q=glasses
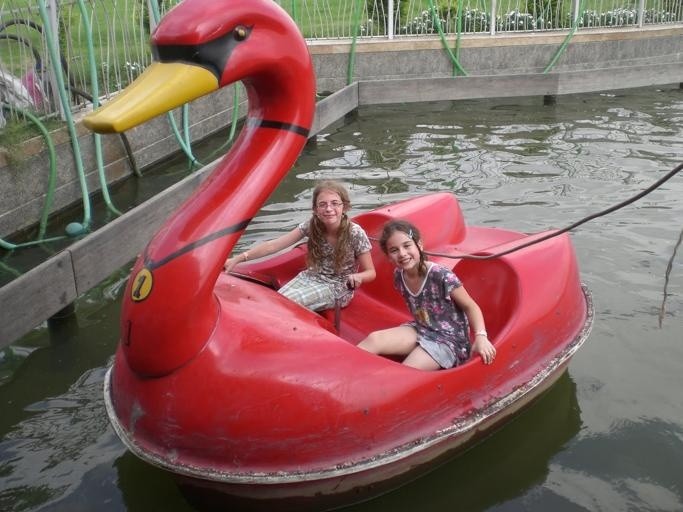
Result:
[315,202,343,209]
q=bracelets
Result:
[474,330,488,336]
[241,251,248,262]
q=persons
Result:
[223,178,378,312]
[356,220,497,368]
